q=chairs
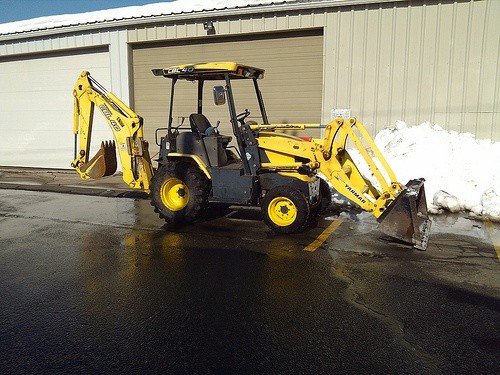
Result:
[189,114,233,147]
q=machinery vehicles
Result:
[69,58,433,253]
